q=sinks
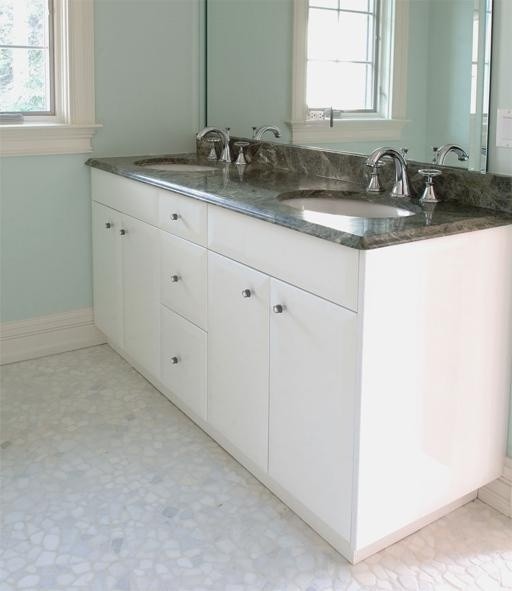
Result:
[276,189,423,218]
[133,156,226,172]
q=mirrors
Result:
[204,0,494,177]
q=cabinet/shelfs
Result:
[90,166,511,565]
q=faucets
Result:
[252,124,281,140]
[195,127,232,162]
[432,144,470,165]
[363,145,413,196]
